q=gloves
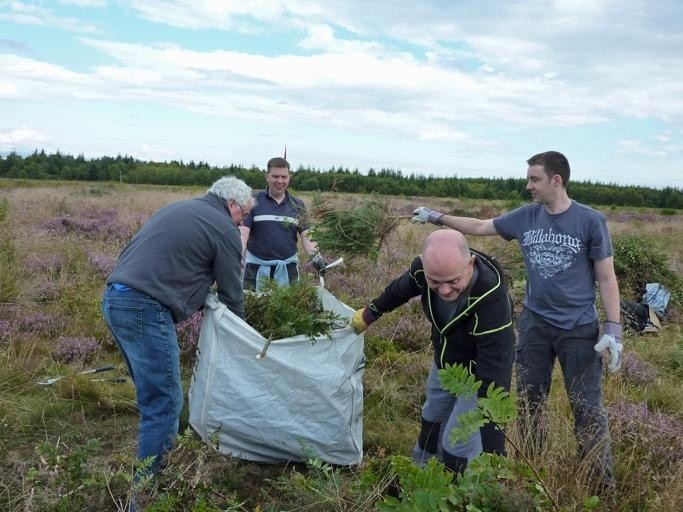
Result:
[593,321,623,374]
[411,207,443,226]
[309,251,330,277]
[351,305,379,335]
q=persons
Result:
[348,227,518,473]
[226,157,324,300]
[98,172,259,491]
[409,150,626,501]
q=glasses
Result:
[233,198,248,220]
